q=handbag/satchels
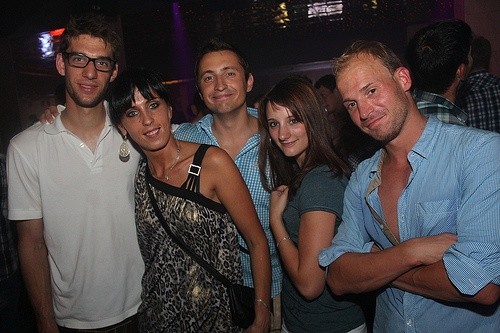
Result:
[227,283,258,328]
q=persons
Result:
[405,18,500,134]
[6,15,180,333]
[189,91,209,124]
[319,40,500,333]
[0,153,40,331]
[252,96,269,108]
[315,75,369,164]
[260,76,368,333]
[39,41,285,333]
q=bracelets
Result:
[276,235,293,247]
[256,296,270,312]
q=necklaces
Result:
[147,139,180,182]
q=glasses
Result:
[61,50,117,72]
